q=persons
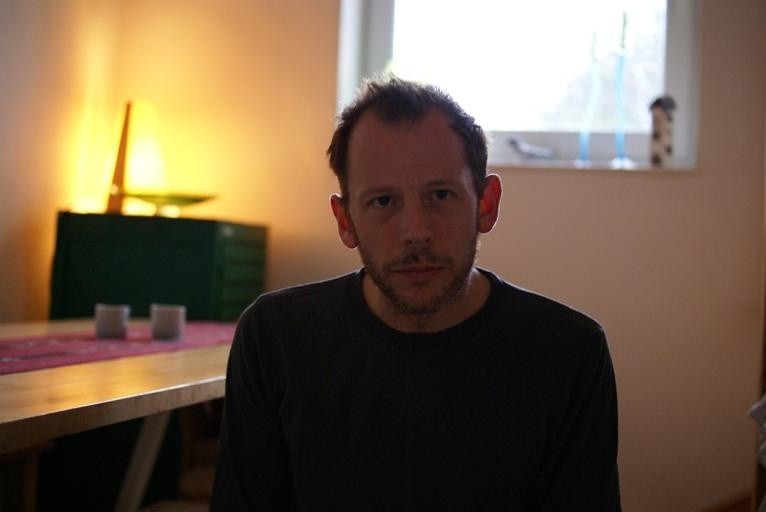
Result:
[209,77,620,512]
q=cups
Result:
[93,304,129,337]
[151,302,186,339]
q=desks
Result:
[1,314,235,512]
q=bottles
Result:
[650,96,676,164]
